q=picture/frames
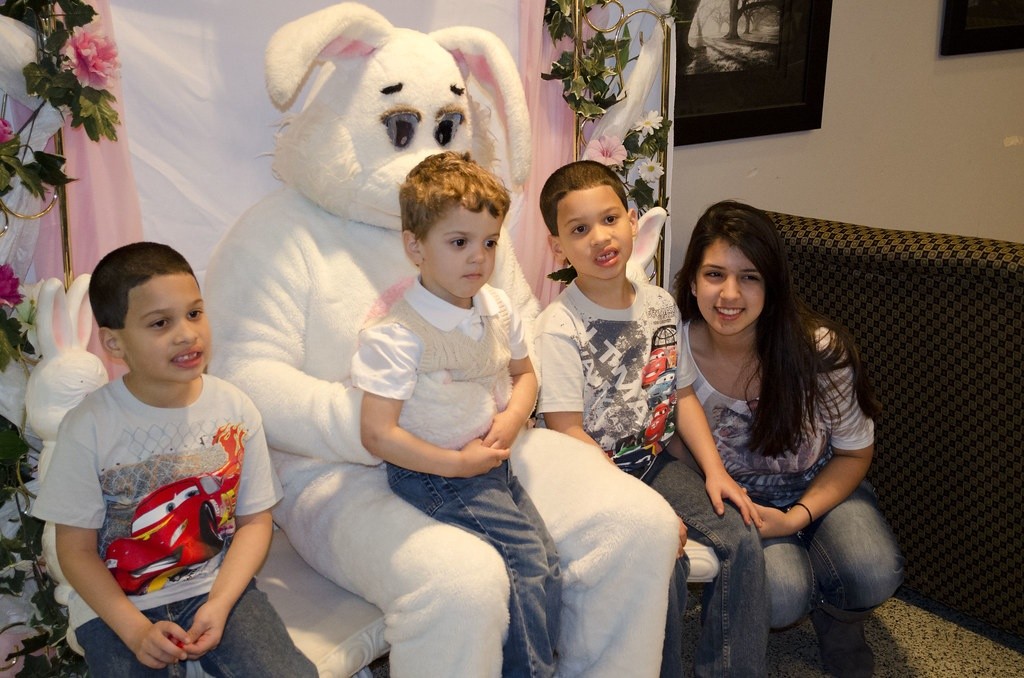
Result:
[665,0,833,147]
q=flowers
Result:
[541,0,674,218]
[0,0,121,678]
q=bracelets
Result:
[786,502,813,529]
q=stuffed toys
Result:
[26,274,109,606]
[206,1,678,678]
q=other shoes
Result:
[810,608,874,678]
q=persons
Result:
[679,197,904,678]
[30,242,321,678]
[353,148,563,678]
[531,161,773,678]
